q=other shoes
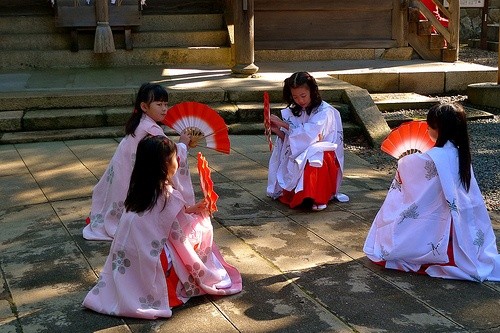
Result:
[312,203,328,211]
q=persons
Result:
[82,82,196,241]
[82,134,243,320]
[362,101,500,283]
[266,72,349,211]
